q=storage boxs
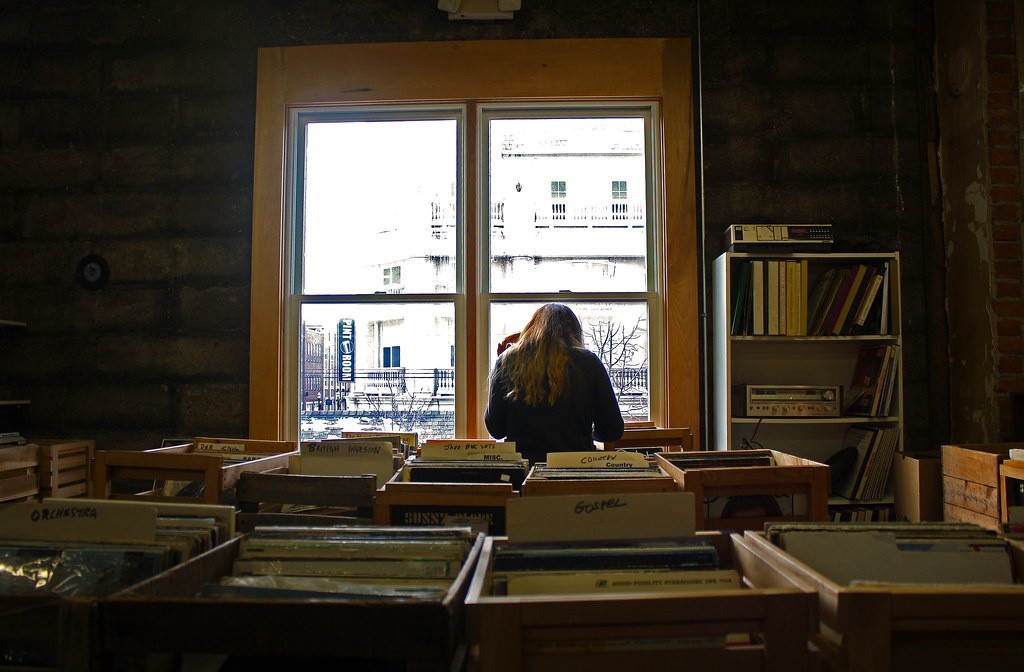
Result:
[0,437,1024,672]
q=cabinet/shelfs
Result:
[712,251,905,523]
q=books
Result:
[763,522,1017,587]
[727,259,900,523]
[0,435,777,602]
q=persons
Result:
[484,304,625,470]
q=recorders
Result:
[745,384,841,418]
